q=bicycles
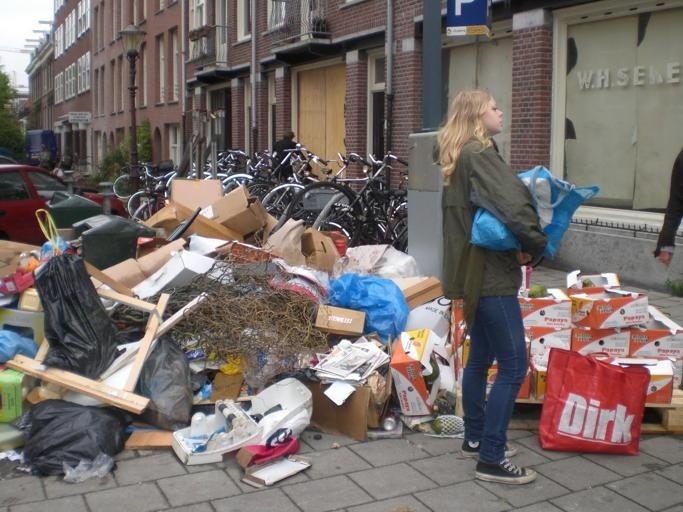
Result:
[113,142,408,253]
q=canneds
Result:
[382,409,395,431]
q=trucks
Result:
[24,128,56,166]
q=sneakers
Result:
[461,439,517,457]
[475,459,537,485]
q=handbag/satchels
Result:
[469,165,599,261]
[539,346,651,456]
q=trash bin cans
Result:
[45,191,156,272]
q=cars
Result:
[0,156,17,165]
[0,164,127,246]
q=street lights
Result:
[119,24,146,221]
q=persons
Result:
[273,131,311,183]
[62,148,80,171]
[653,149,682,267]
[433,91,547,486]
[38,147,52,171]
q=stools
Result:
[0,308,47,351]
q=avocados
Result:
[584,279,595,287]
[527,284,547,298]
[433,415,465,435]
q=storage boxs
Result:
[166,174,273,245]
[312,303,366,337]
[387,271,683,430]
[296,229,342,272]
[1,368,34,423]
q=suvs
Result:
[0,147,39,166]
